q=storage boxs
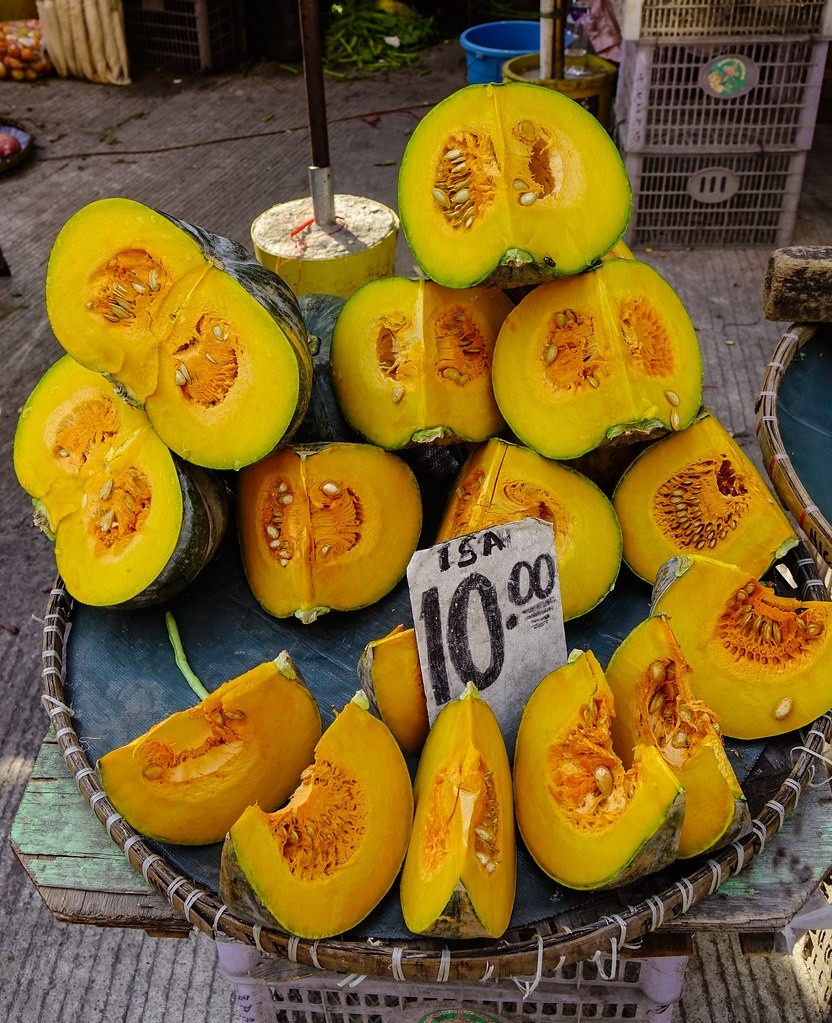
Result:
[614,0,832,249]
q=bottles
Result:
[563,1,589,78]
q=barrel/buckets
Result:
[458,21,577,86]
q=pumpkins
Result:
[94,411,829,945]
[13,83,704,615]
